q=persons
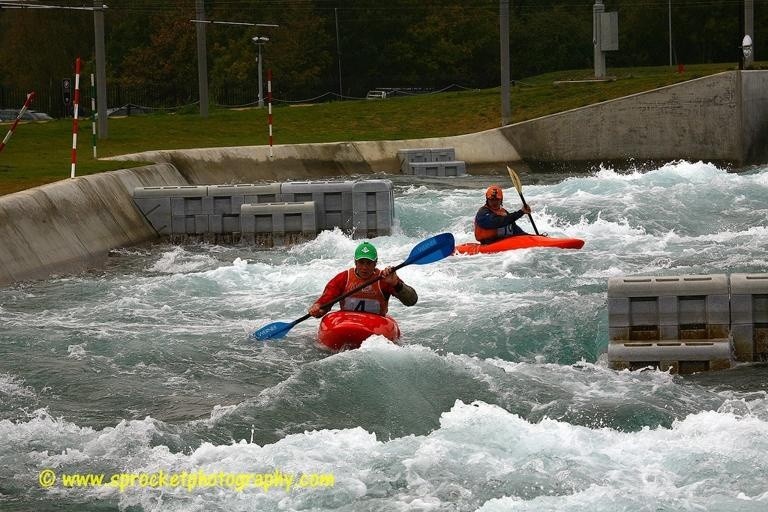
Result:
[474,186,548,245]
[309,242,418,318]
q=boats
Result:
[319,310,400,351]
[456,234,585,256]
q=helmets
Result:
[486,186,503,201]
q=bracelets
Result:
[394,281,403,293]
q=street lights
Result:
[252,36,270,109]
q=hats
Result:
[355,242,378,262]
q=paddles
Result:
[251,233,454,339]
[506,166,539,235]
